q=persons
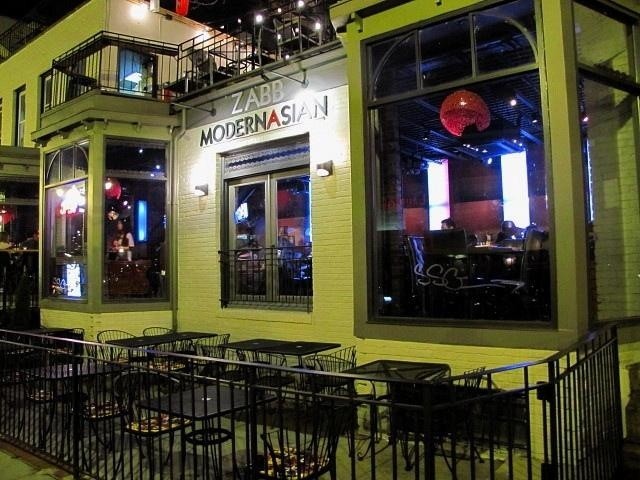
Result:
[300,242,312,259]
[496,221,525,243]
[114,219,136,261]
[0,230,12,250]
[18,228,39,300]
[441,218,456,230]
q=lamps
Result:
[195,183,208,200]
[315,158,333,178]
[440,88,493,139]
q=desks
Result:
[339,359,453,473]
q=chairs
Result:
[379,226,550,318]
[162,1,331,90]
[0,324,501,479]
